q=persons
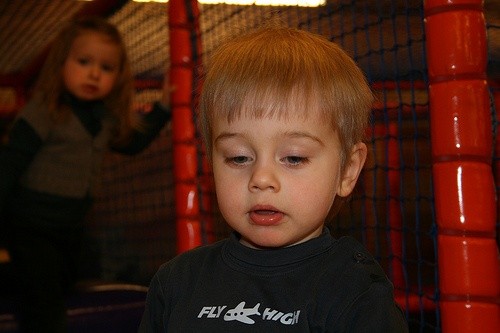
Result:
[0,16,171,333]
[134,26,405,332]
